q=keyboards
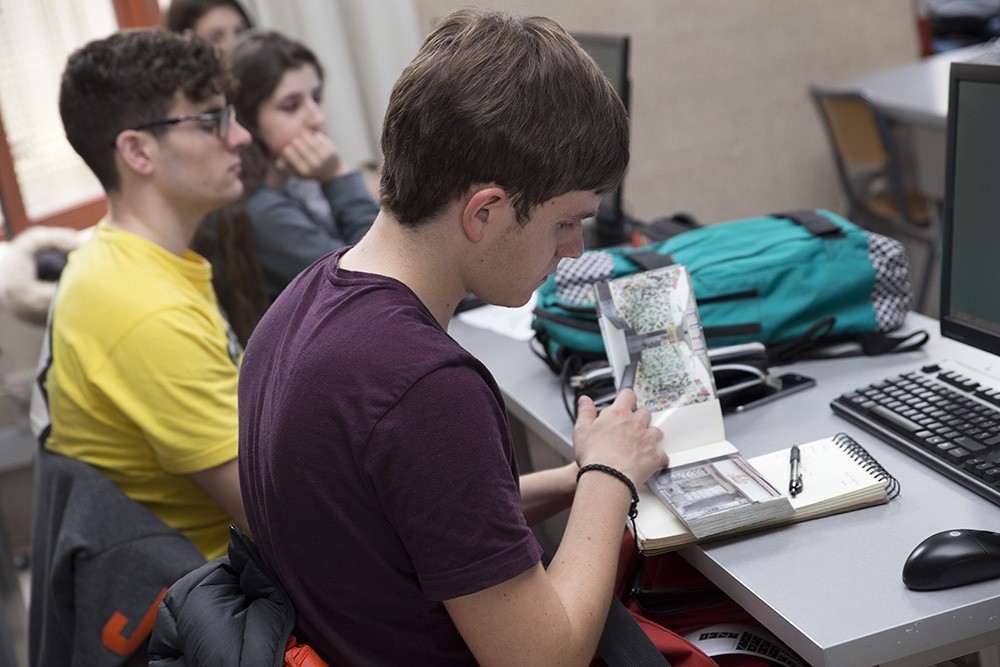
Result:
[829,363,1000,508]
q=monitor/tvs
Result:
[572,29,632,230]
[939,63,1000,358]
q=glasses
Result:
[110,105,234,148]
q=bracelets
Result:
[576,463,640,566]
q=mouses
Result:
[901,528,1000,593]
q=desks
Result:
[446,287,1000,667]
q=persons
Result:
[214,29,381,351]
[29,25,254,562]
[236,10,669,667]
[166,0,252,48]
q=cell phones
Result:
[719,374,816,414]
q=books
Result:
[624,431,900,558]
[592,262,798,539]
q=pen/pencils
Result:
[789,446,804,497]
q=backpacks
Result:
[529,209,930,375]
